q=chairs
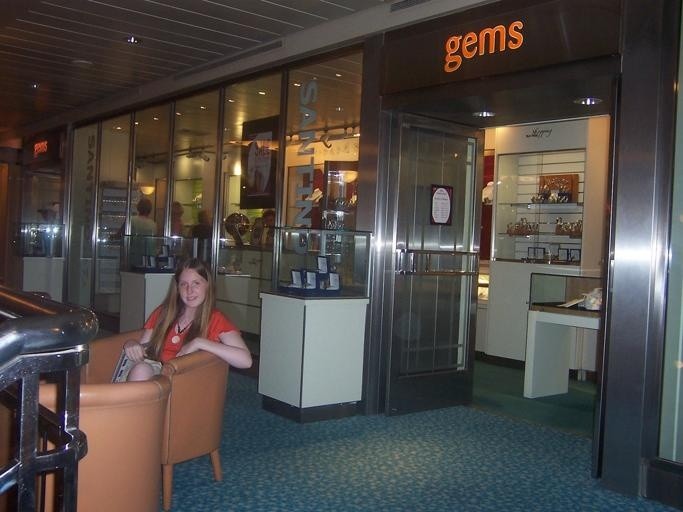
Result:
[38,327,230,511]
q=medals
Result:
[170,335,180,345]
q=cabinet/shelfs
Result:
[319,159,358,232]
[493,148,586,268]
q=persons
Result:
[187,210,212,240]
[261,209,275,246]
[118,199,158,237]
[248,217,263,245]
[171,201,184,236]
[110,256,253,384]
